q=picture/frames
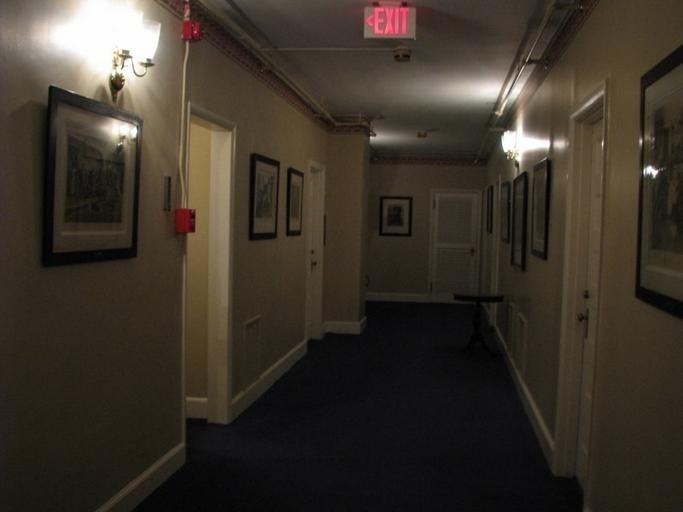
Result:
[285,167,304,237]
[41,82,144,269]
[632,42,681,322]
[247,154,281,243]
[485,157,553,270]
[376,194,414,238]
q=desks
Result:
[450,288,507,357]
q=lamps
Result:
[498,129,519,170]
[87,16,161,103]
